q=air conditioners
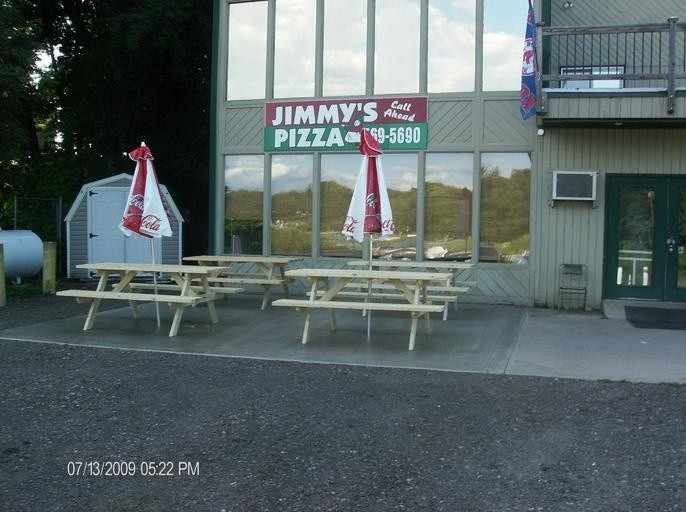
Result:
[547,170,599,209]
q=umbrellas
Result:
[118,141,174,329]
[341,130,396,339]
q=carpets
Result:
[624,306,686,330]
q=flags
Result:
[520,4,538,121]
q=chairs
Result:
[558,263,587,314]
